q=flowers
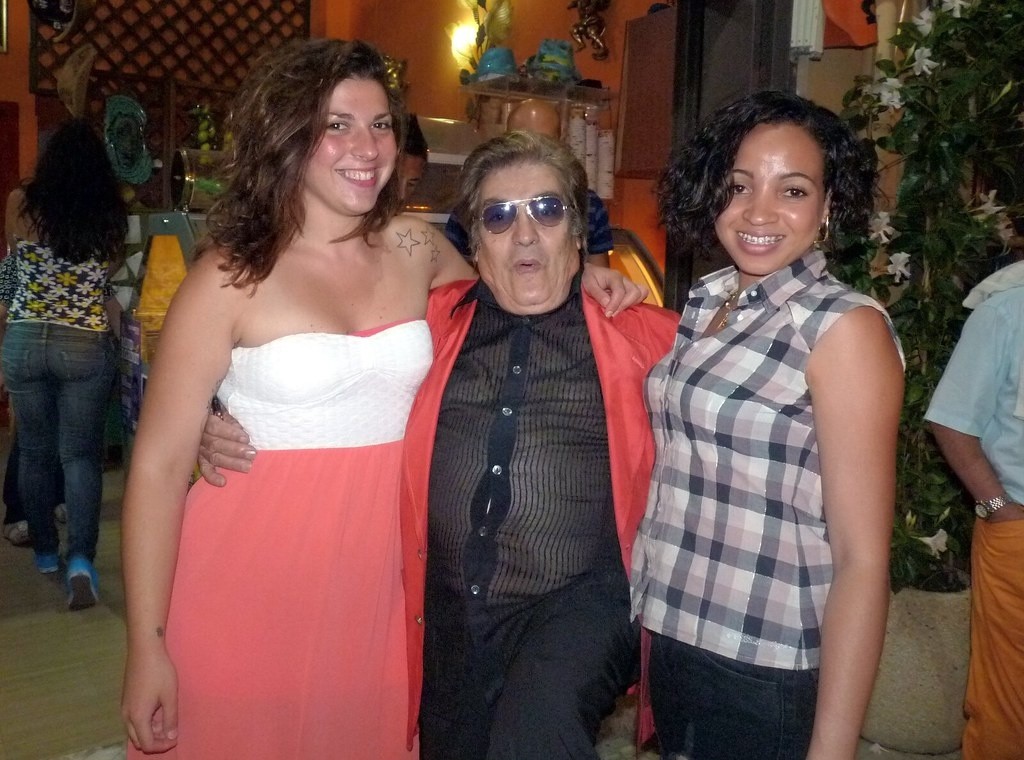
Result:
[824,0,1024,593]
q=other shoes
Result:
[56,504,70,524]
[2,519,30,547]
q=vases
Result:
[859,586,971,755]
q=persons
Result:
[388,114,428,215]
[0,120,130,608]
[445,96,615,267]
[196,129,683,760]
[118,37,648,760]
[629,87,905,760]
[923,259,1024,760]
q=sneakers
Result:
[34,553,62,573]
[64,556,98,611]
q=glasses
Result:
[474,194,581,235]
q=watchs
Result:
[975,493,1011,520]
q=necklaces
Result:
[717,292,738,332]
